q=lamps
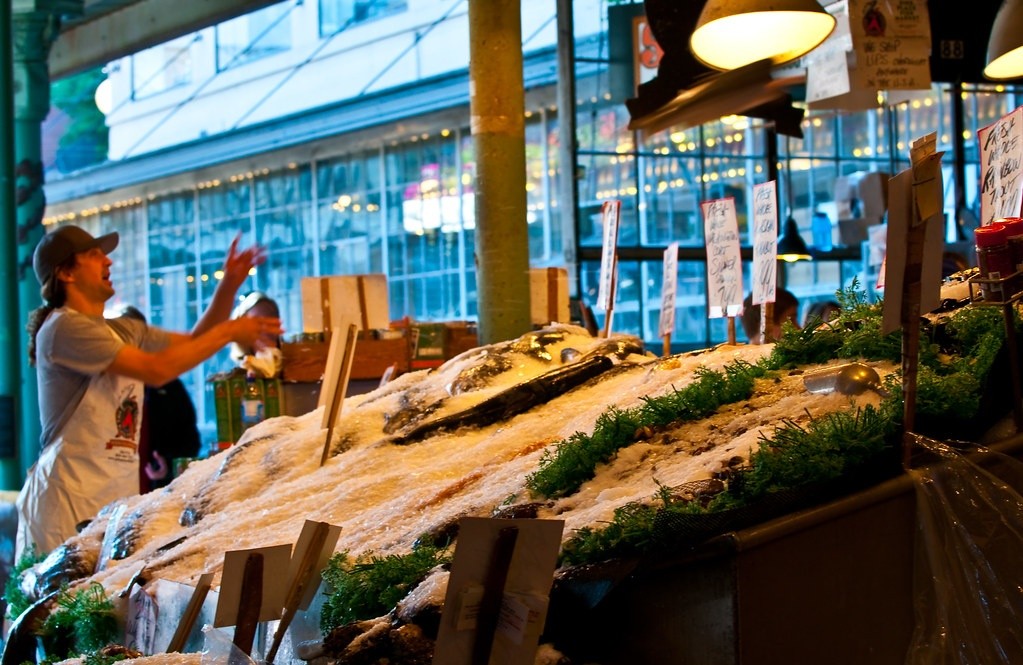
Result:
[688,0,837,72]
[982,0,1023,81]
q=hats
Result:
[32,224,120,285]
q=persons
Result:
[234,292,285,356]
[800,300,840,328]
[740,285,803,346]
[107,301,203,496]
[15,224,285,567]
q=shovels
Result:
[802,362,893,400]
